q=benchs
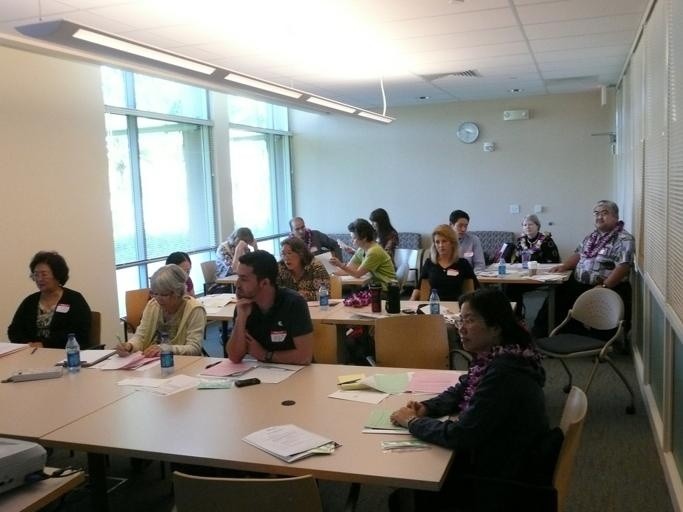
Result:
[320,233,418,258]
[464,230,514,264]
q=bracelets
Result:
[600,283,607,289]
[407,414,416,425]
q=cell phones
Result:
[403,308,415,314]
[235,377,260,387]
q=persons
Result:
[6,251,92,349]
[549,199,636,336]
[390,287,558,512]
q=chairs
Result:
[119,260,516,367]
[171,470,324,512]
[531,287,637,414]
[547,386,588,512]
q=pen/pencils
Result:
[31,347,37,354]
[205,360,222,369]
[117,336,130,355]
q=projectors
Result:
[0,436,47,494]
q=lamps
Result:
[13,1,398,125]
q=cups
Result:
[526,261,538,276]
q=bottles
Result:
[318,282,329,309]
[429,289,440,315]
[369,281,383,313]
[498,255,506,277]
[385,280,403,313]
[65,333,81,374]
[521,246,530,268]
[158,332,175,376]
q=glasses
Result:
[454,318,487,328]
[592,211,614,216]
[151,292,174,300]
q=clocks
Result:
[455,121,480,145]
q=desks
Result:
[0,466,86,512]
[0,339,204,512]
[38,355,471,511]
[474,258,635,334]
